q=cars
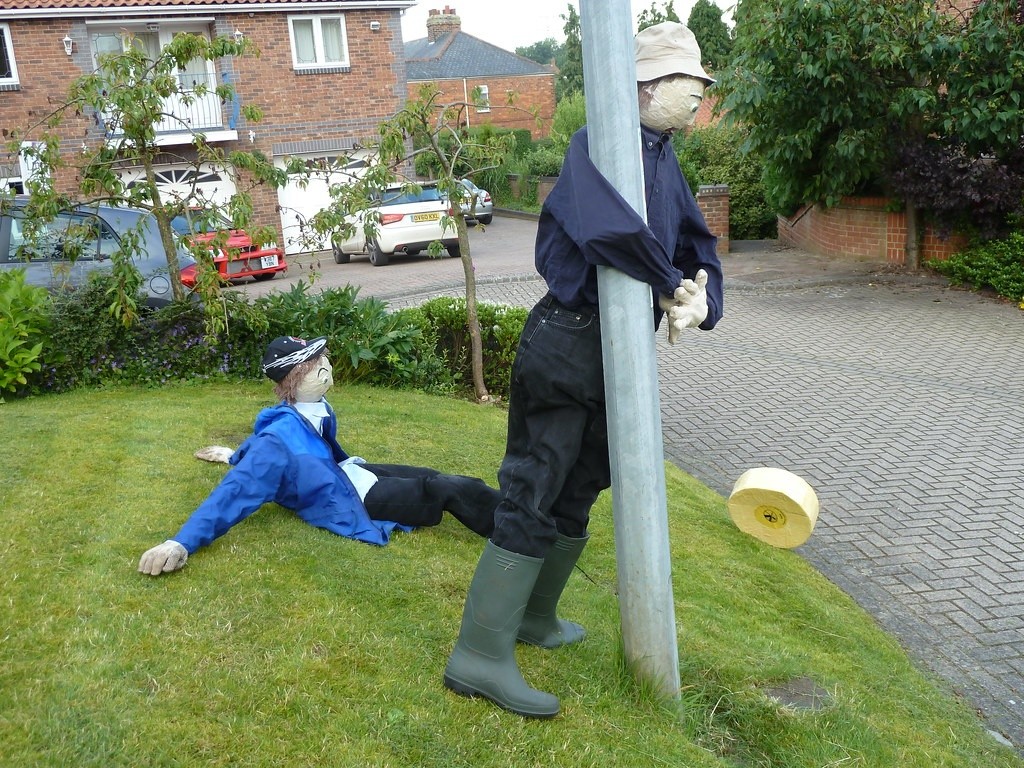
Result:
[331,182,463,266]
[453,178,492,226]
[163,206,287,288]
[0,194,210,323]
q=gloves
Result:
[659,278,698,344]
[137,540,189,575]
[194,446,236,464]
[670,269,708,331]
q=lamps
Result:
[233,29,244,44]
[62,34,73,56]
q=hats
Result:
[635,19,717,89]
[262,335,327,382]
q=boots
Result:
[516,531,592,650]
[446,538,562,720]
